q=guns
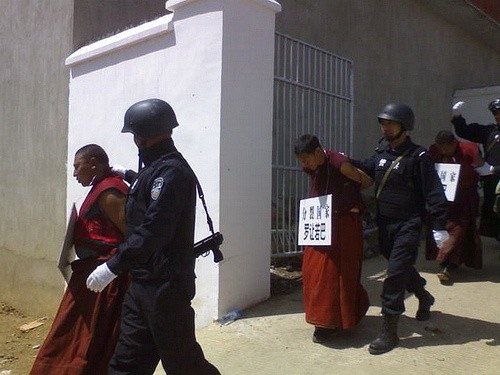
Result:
[192,232,225,263]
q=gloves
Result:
[86,263,118,293]
[110,163,128,179]
[433,230,449,249]
[475,162,494,176]
[452,101,465,116]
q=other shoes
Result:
[438,268,452,282]
[312,326,331,342]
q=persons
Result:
[451,97,500,242]
[85,98,221,375]
[293,134,374,344]
[424,129,490,284]
[336,103,451,354]
[28,144,133,375]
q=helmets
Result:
[121,98,179,142]
[377,103,414,131]
[487,97,500,109]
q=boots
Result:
[413,287,435,321]
[368,314,399,354]
